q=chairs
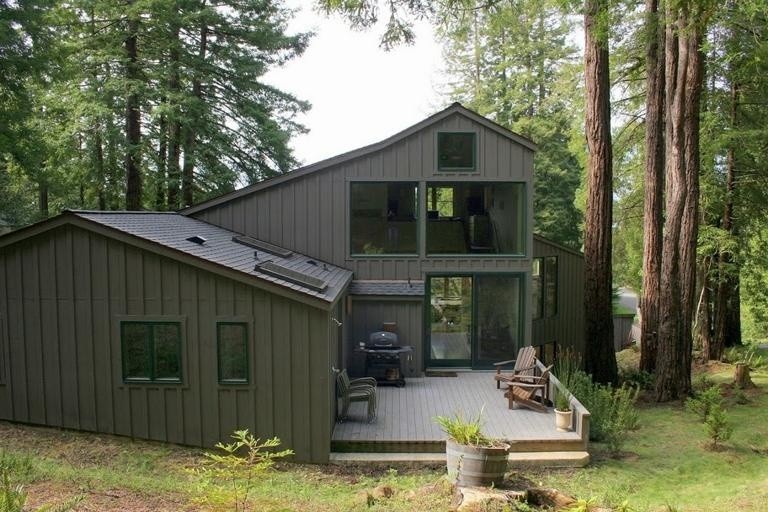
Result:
[337,369,378,423]
[492,345,538,390]
[503,364,554,413]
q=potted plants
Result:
[435,414,511,487]
[553,389,573,428]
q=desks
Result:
[470,245,490,253]
[354,346,412,387]
